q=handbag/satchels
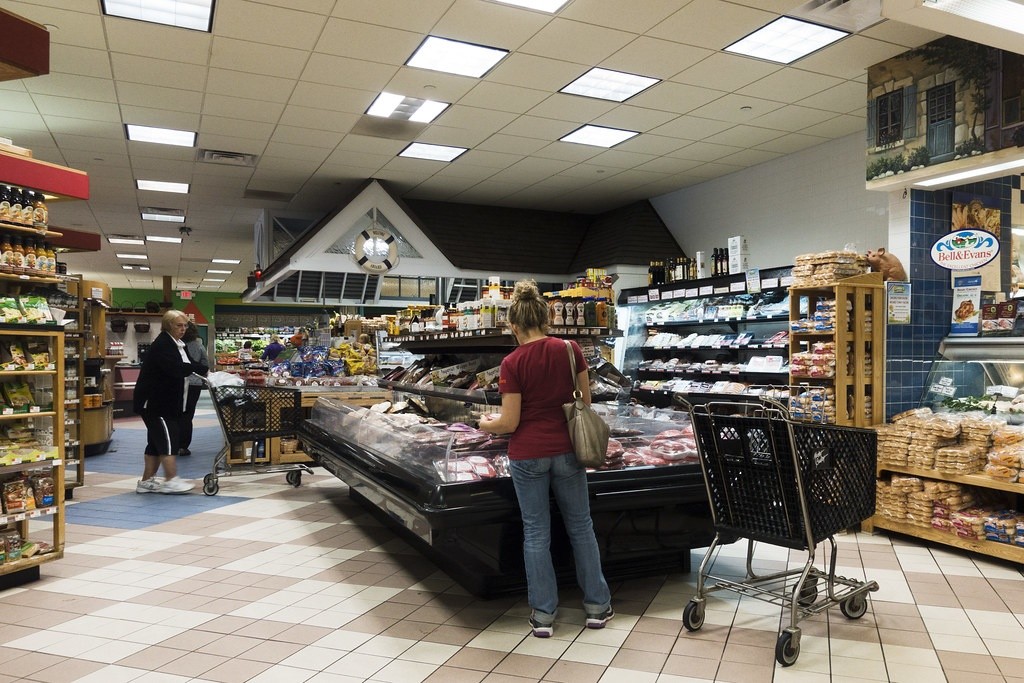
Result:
[562,339,610,467]
[208,371,259,407]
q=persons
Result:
[133,309,210,494]
[180,321,209,456]
[478,281,617,637]
[262,336,286,361]
[240,340,253,351]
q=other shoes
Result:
[179,449,191,456]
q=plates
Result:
[403,395,427,415]
[377,400,408,413]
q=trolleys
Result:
[673,384,880,667]
[192,371,317,496]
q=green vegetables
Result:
[216,339,267,357]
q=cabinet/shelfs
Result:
[782,272,887,536]
[861,458,1024,566]
[378,327,628,428]
[0,5,126,592]
[628,313,807,401]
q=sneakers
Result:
[136,476,162,493]
[529,618,553,637]
[158,477,196,493]
[586,605,615,629]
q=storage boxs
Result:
[696,250,705,278]
[728,236,750,276]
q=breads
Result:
[864,406,1024,549]
[789,251,872,425]
[952,204,1000,241]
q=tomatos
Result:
[218,357,241,365]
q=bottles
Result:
[648,256,697,286]
[0,233,55,273]
[394,302,457,335]
[541,295,615,328]
[0,184,48,230]
[711,248,729,277]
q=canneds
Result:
[47,294,79,309]
